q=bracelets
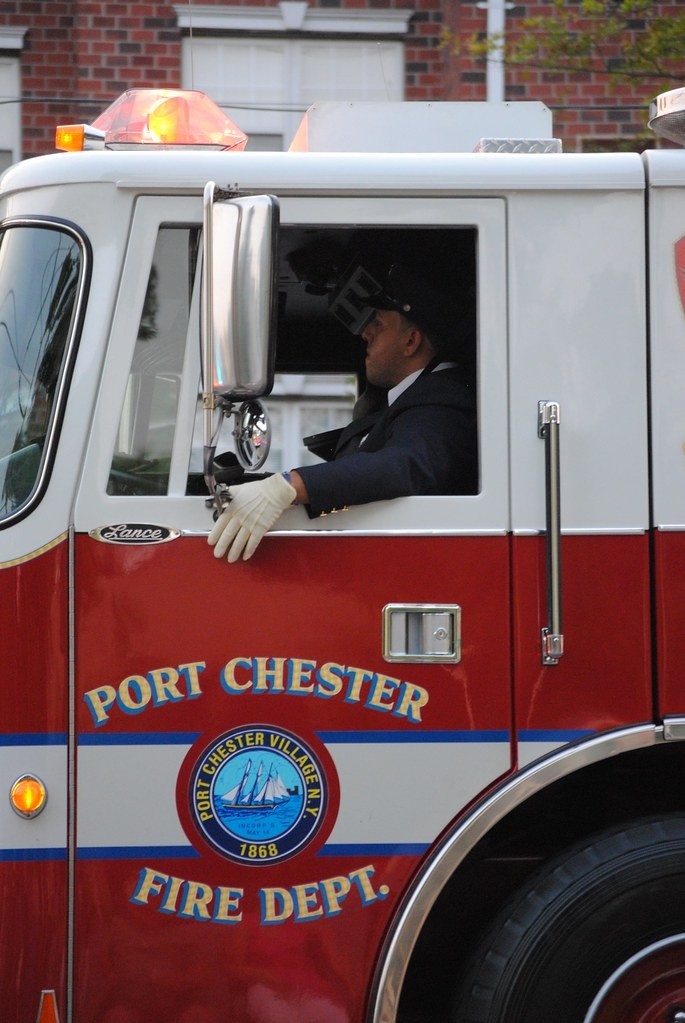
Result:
[282,472,299,505]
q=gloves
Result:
[208,471,297,563]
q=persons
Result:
[206,261,478,563]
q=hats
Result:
[363,267,461,361]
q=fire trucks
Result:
[0,86,685,1023]
